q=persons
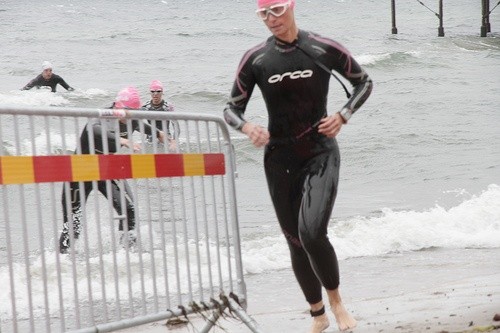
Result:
[131,78,181,147]
[19,60,76,94]
[222,0,377,333]
[59,88,176,253]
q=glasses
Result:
[255,0,292,21]
[150,90,162,94]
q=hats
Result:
[149,80,163,92]
[114,87,141,109]
[257,0,296,12]
[41,61,53,73]
